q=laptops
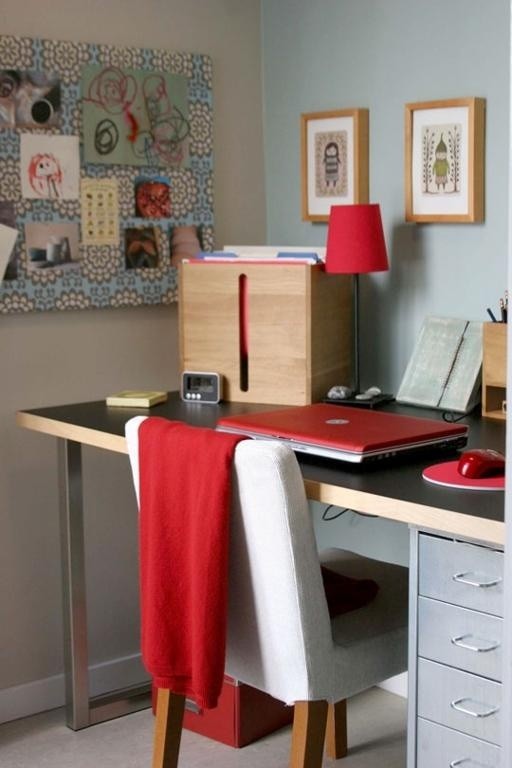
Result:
[216,402,470,466]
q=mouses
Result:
[452,446,499,478]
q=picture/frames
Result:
[300,97,484,224]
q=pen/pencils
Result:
[487,289,508,323]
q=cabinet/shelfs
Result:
[13,391,506,768]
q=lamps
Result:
[319,204,395,409]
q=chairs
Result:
[124,417,409,768]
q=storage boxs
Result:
[481,323,506,418]
[178,256,357,407]
